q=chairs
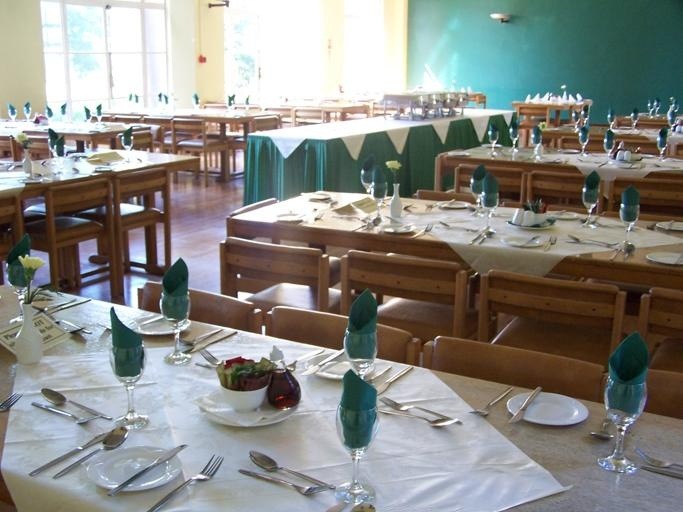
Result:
[424,335,606,400]
[644,367,683,419]
[0,99,683,367]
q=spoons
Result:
[588,419,613,437]
[378,409,459,427]
[623,243,635,260]
[53,426,129,480]
[352,217,381,232]
[248,449,335,490]
[39,387,112,419]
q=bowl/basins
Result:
[217,379,267,412]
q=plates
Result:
[302,193,330,201]
[656,221,682,231]
[646,251,682,266]
[200,388,297,428]
[506,391,589,425]
[271,211,306,223]
[305,352,373,379]
[482,144,500,148]
[128,316,190,335]
[88,446,182,493]
[447,151,469,156]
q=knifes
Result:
[373,365,413,395]
[28,432,105,476]
[327,500,346,512]
[638,465,683,480]
[300,349,345,376]
[195,361,217,367]
[189,331,237,352]
[109,443,189,496]
[508,385,542,424]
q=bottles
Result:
[267,360,301,411]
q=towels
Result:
[606,329,650,415]
[339,368,378,450]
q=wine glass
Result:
[160,290,190,364]
[335,402,379,502]
[47,137,134,174]
[596,376,646,474]
[106,342,149,430]
[343,328,377,380]
[1,265,32,323]
[359,168,640,246]
[8,108,103,131]
[487,101,678,161]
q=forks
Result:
[634,447,682,470]
[31,305,93,334]
[197,347,225,365]
[178,328,222,346]
[285,348,325,370]
[470,386,512,415]
[238,468,331,495]
[0,390,23,412]
[29,401,101,425]
[145,453,223,512]
[378,396,462,424]
